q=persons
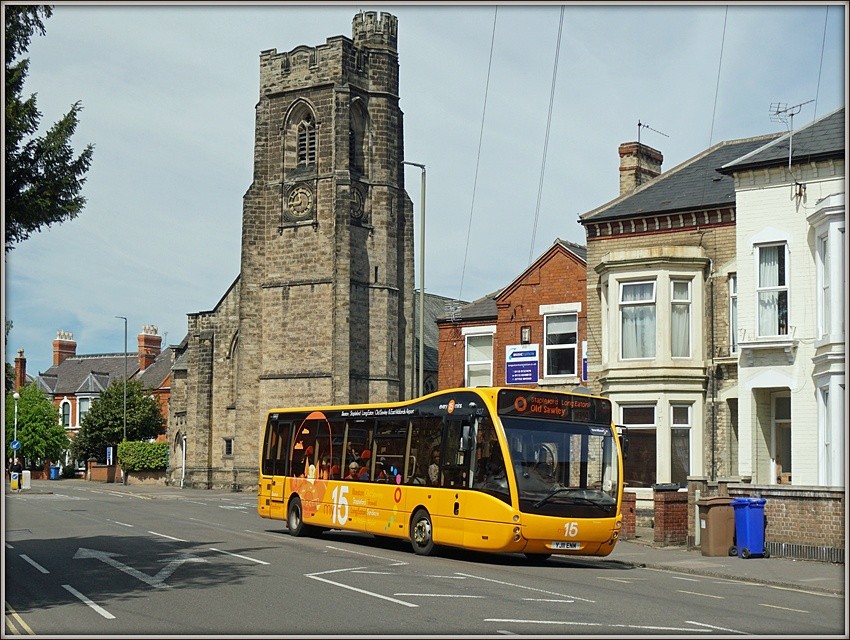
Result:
[426,445,440,486]
[8,458,23,490]
[345,462,360,479]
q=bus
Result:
[257,384,631,564]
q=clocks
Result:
[349,178,374,229]
[278,178,318,236]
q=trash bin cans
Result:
[650,482,680,529]
[697,497,738,557]
[729,497,769,559]
[49,466,60,480]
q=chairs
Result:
[303,446,385,482]
